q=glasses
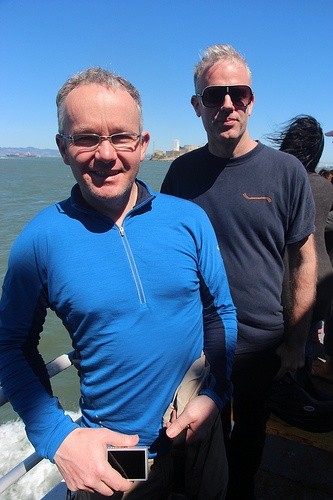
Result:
[60,132,141,149]
[197,86,254,108]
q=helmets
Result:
[264,378,333,431]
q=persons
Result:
[265,115,333,373]
[160,44,318,500]
[1,69,237,500]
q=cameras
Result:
[105,446,148,482]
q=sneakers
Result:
[317,344,330,363]
